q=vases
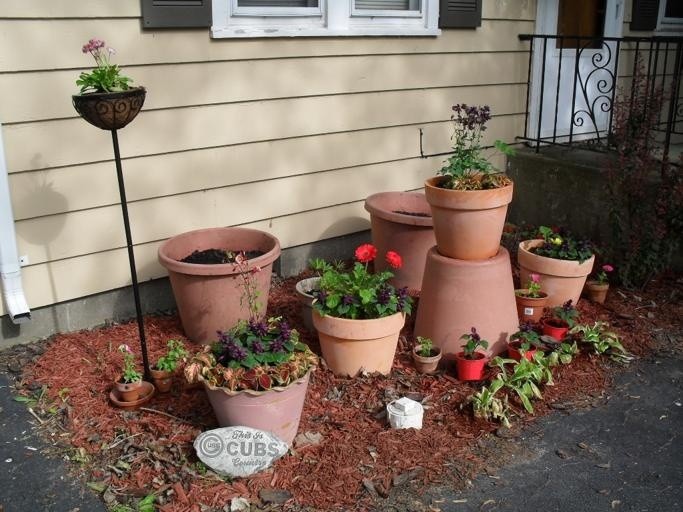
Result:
[506,340,538,362]
[457,351,488,382]
[157,226,282,347]
[412,244,522,362]
[514,288,551,323]
[108,377,153,409]
[71,86,146,130]
[518,239,595,311]
[586,281,609,304]
[311,298,406,380]
[425,176,514,260]
[364,191,436,297]
[295,277,326,335]
[198,355,318,453]
[540,317,570,341]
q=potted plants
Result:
[150,339,187,392]
[412,336,443,373]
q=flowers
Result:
[537,224,592,260]
[509,320,540,351]
[432,101,516,190]
[589,265,613,286]
[183,249,320,392]
[75,36,136,95]
[551,299,580,329]
[458,327,488,359]
[116,343,142,384]
[524,273,542,298]
[306,242,416,318]
